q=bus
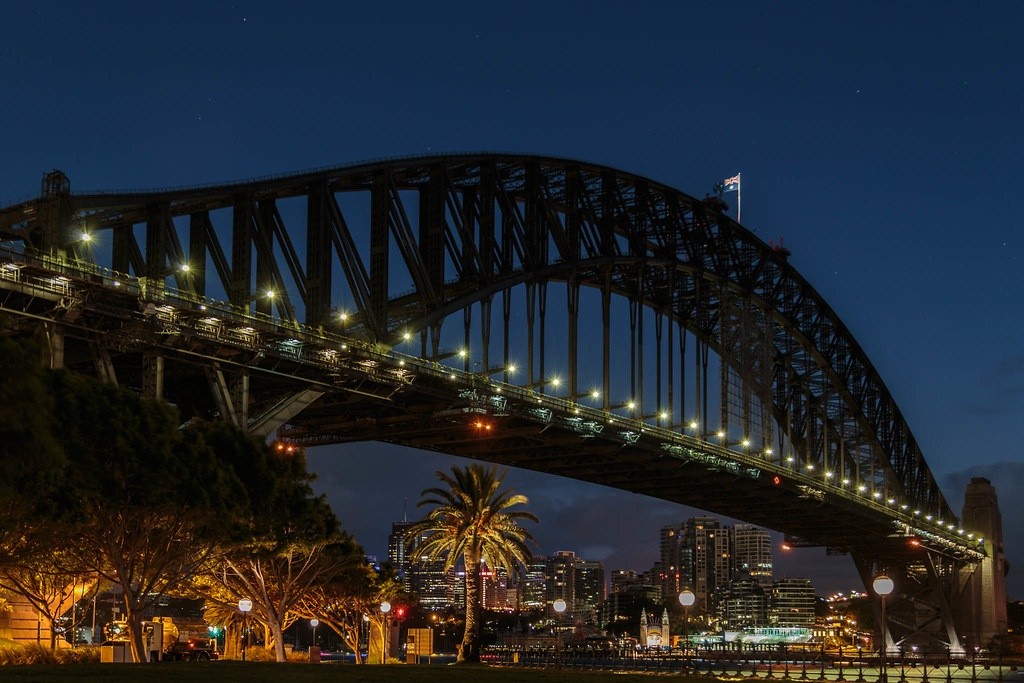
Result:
[740,627,816,645]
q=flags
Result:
[723,175,740,194]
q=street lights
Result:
[553,598,567,669]
[238,598,253,661]
[380,601,392,664]
[872,576,895,683]
[310,618,320,647]
[678,590,696,674]
